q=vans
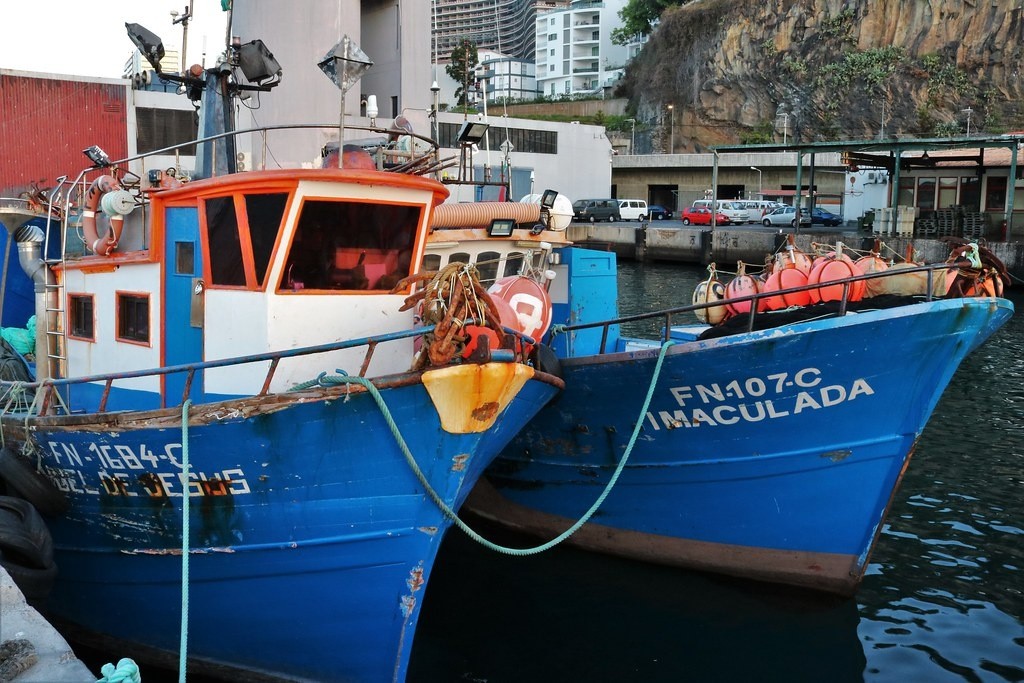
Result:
[737,199,790,224]
[615,199,648,222]
[692,199,751,226]
[572,199,620,223]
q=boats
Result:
[0,122,1014,682]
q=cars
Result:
[643,206,673,220]
[761,207,812,227]
[681,206,731,226]
[800,207,844,227]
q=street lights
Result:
[881,95,885,141]
[668,105,674,154]
[776,103,787,153]
[429,81,441,182]
[962,106,973,138]
[318,35,374,168]
[124,21,284,173]
[624,119,635,155]
[529,170,535,203]
[751,166,761,193]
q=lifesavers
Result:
[81,172,126,255]
